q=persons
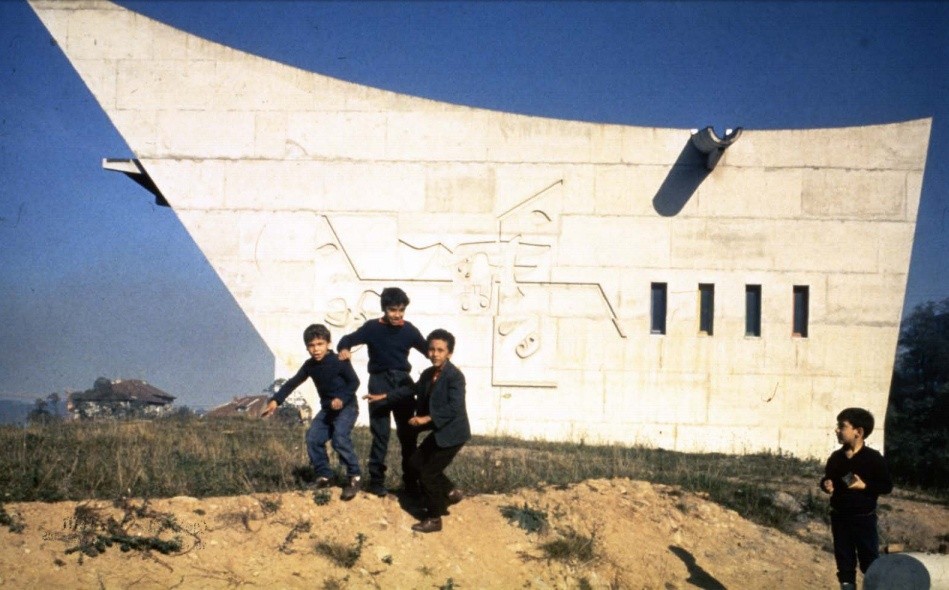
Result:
[259,323,364,500]
[362,328,471,533]
[818,408,893,590]
[336,287,431,498]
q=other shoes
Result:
[443,488,464,509]
[317,478,333,489]
[349,477,364,488]
[411,517,442,532]
